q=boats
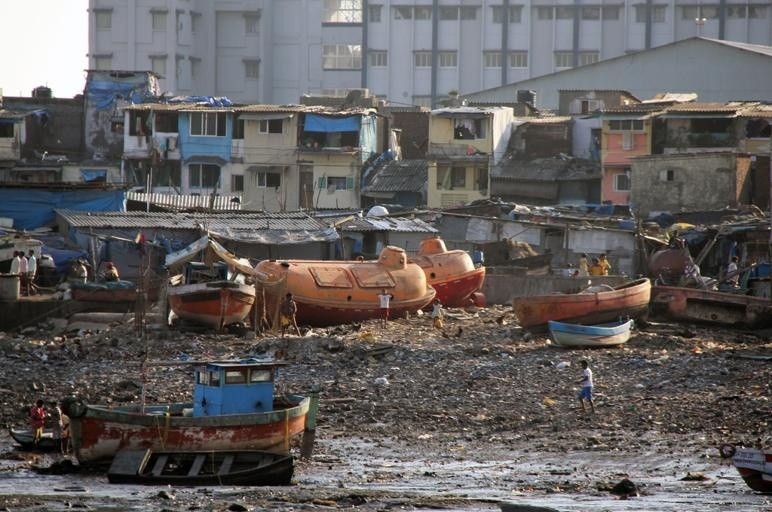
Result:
[60,357,310,470]
[470,239,553,278]
[649,262,771,327]
[67,229,170,302]
[106,447,294,485]
[720,445,771,493]
[546,319,633,348]
[513,278,651,337]
[165,235,256,333]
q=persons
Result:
[11,248,123,294]
[430,298,444,331]
[726,256,742,286]
[376,287,393,330]
[575,359,597,416]
[668,230,686,249]
[31,399,46,445]
[472,246,486,264]
[567,252,612,277]
[60,394,87,466]
[279,292,304,337]
[51,401,64,457]
